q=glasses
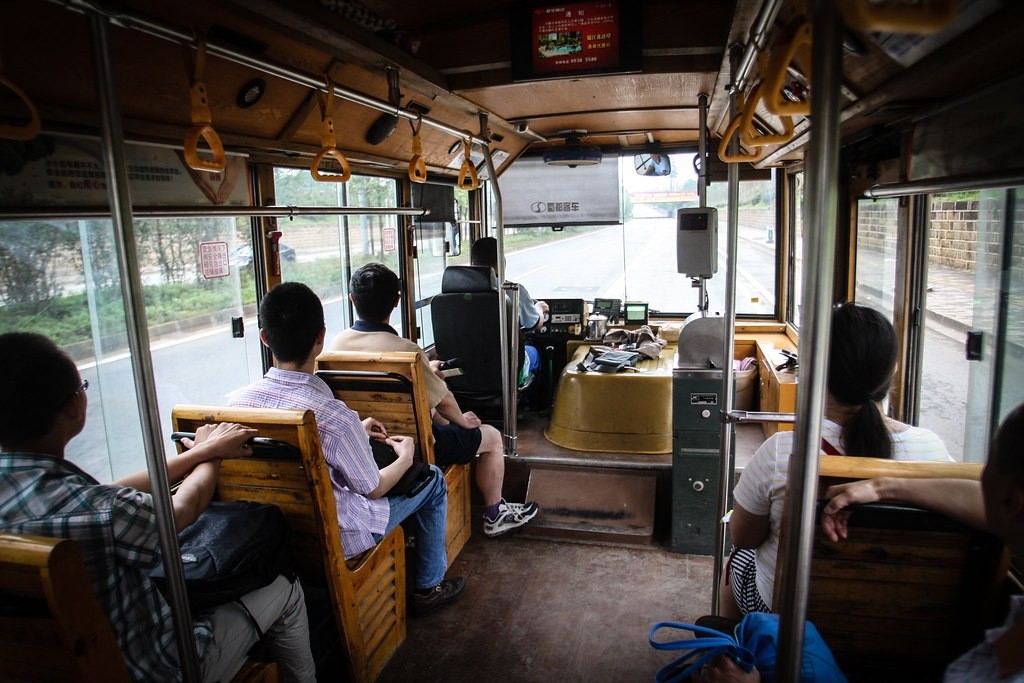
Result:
[73,378,88,396]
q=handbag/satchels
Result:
[649,612,849,683]
[367,438,436,498]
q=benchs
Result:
[779,482,1002,683]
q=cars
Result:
[227,238,295,272]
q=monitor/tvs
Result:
[624,304,648,325]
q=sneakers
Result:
[483,496,539,538]
[410,577,466,617]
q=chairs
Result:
[0,534,279,682]
[171,404,410,683]
[429,264,547,425]
[313,351,471,576]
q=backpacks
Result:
[148,498,289,616]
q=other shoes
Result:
[694,616,739,639]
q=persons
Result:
[0,332,315,683]
[717,303,952,620]
[330,263,539,538]
[643,154,669,175]
[692,400,1024,683]
[222,281,468,618]
[470,237,550,387]
